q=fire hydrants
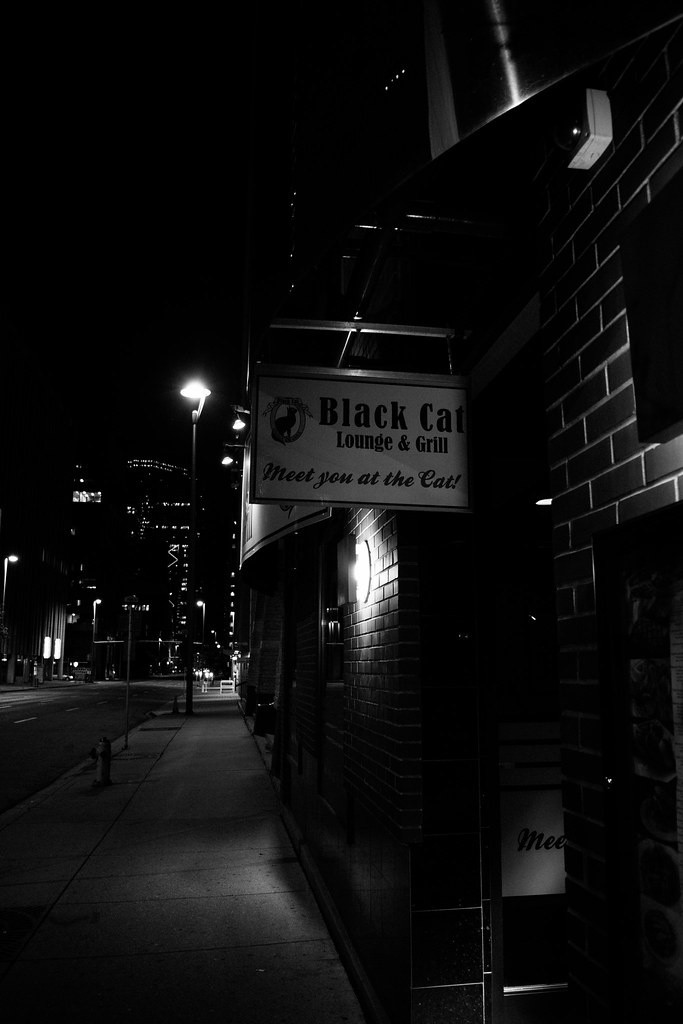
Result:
[92,737,113,786]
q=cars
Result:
[52,672,73,681]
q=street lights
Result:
[3,555,19,615]
[89,598,102,683]
[196,600,206,682]
[179,378,212,718]
